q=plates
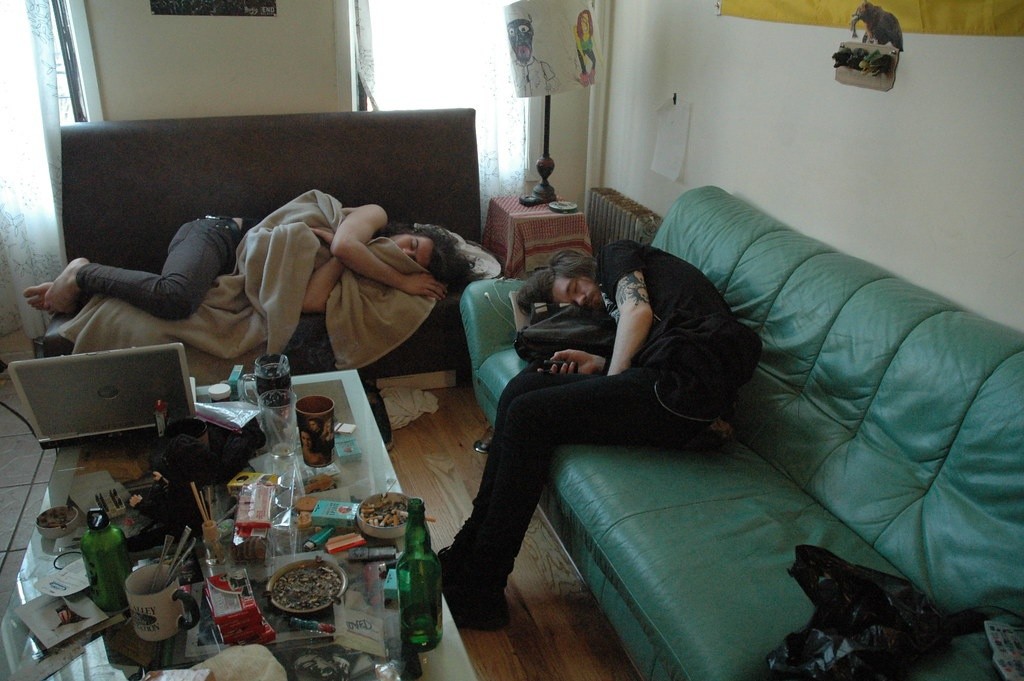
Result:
[265,559,349,612]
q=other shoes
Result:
[366,389,394,450]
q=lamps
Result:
[495,0,603,205]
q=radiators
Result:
[586,185,663,262]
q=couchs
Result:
[37,105,486,395]
[453,183,1024,681]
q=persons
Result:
[22,204,488,321]
[438,240,740,631]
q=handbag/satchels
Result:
[514,304,617,363]
[765,545,953,681]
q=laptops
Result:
[7,342,196,450]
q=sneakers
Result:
[437,545,512,631]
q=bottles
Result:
[79,508,134,612]
[198,530,229,579]
[396,498,443,646]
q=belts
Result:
[223,219,242,247]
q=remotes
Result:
[984,620,1024,681]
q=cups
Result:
[182,419,210,452]
[207,384,231,403]
[294,394,335,468]
[125,564,200,642]
[257,389,297,458]
[238,353,291,405]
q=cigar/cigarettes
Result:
[361,500,436,527]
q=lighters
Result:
[348,546,397,562]
[303,526,334,550]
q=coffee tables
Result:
[0,366,479,681]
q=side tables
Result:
[484,179,594,271]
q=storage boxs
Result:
[205,588,264,631]
[205,567,258,625]
[333,431,362,464]
[311,500,358,528]
[226,469,278,496]
[229,607,275,648]
[383,569,411,600]
[206,596,266,645]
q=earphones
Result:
[496,277,506,281]
[484,292,491,302]
